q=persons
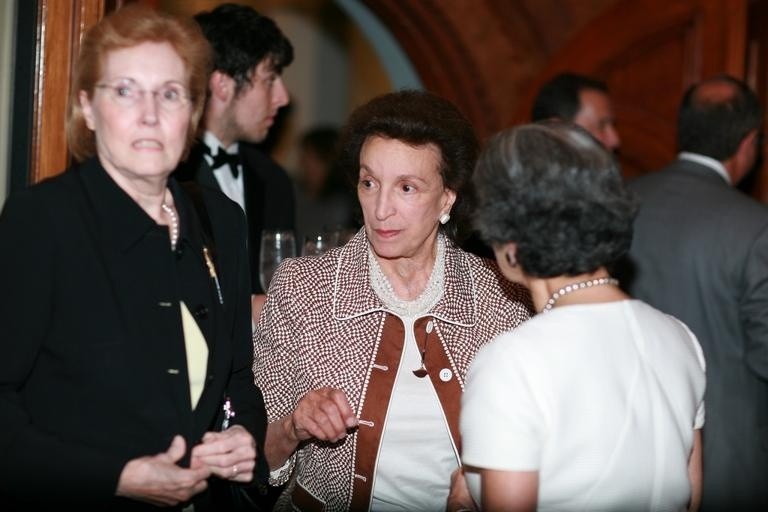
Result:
[529,69,621,156]
[300,122,351,228]
[258,98,295,228]
[624,68,767,512]
[171,2,295,329]
[252,88,537,511]
[1,2,272,511]
[445,118,709,512]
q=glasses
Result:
[93,73,199,115]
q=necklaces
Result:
[159,200,179,253]
[366,230,445,317]
[540,277,620,314]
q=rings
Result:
[231,464,237,476]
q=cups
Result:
[256,226,342,299]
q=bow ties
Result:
[199,141,244,179]
